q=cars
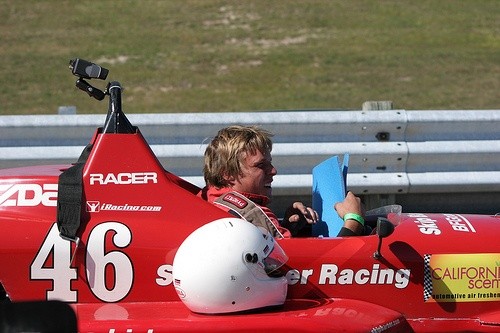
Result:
[0,57,500,333]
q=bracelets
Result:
[343,214,364,226]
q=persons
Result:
[196,125,364,239]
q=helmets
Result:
[172,217,289,314]
